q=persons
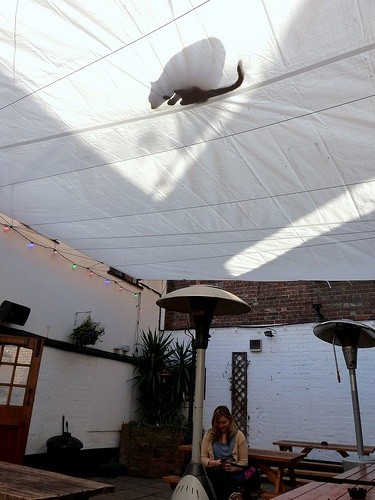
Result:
[200,406,262,500]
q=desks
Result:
[0,461,115,500]
[169,444,307,494]
[268,481,375,500]
[331,460,375,486]
[272,439,375,478]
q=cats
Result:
[145,34,246,109]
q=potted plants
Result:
[70,314,106,348]
[119,326,197,479]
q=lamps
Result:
[264,330,274,338]
[0,299,32,327]
[312,318,375,472]
[155,284,252,500]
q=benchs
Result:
[298,460,344,470]
[162,474,281,500]
[259,473,315,484]
[270,466,340,478]
